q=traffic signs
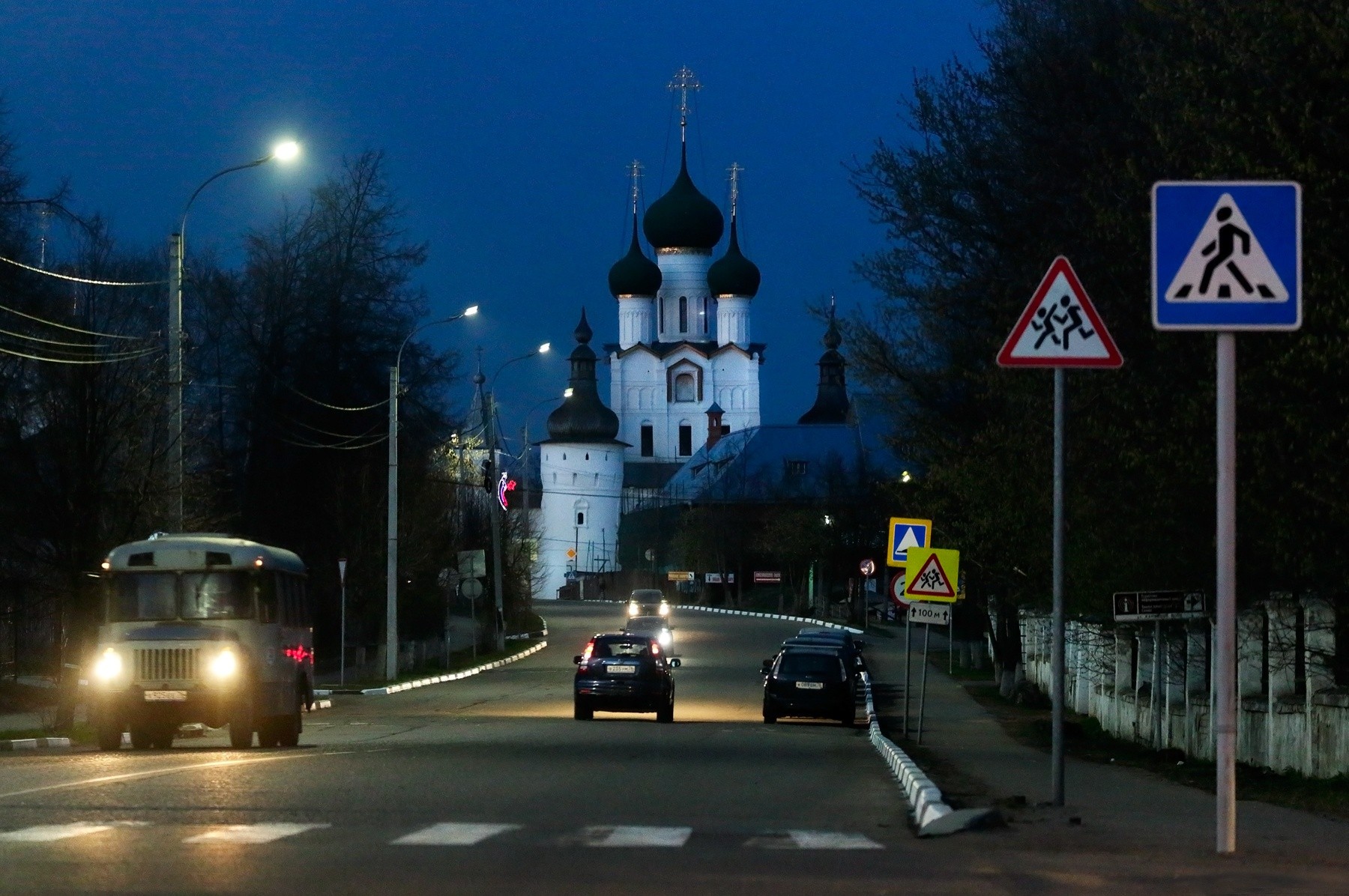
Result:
[909,601,951,627]
[1112,588,1213,624]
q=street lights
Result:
[472,342,551,650]
[523,386,575,613]
[166,138,303,531]
[379,304,480,679]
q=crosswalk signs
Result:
[1150,182,1302,333]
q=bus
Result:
[83,533,322,750]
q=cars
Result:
[762,648,862,727]
[763,627,867,674]
[618,615,677,657]
[624,587,670,625]
[572,632,682,715]
